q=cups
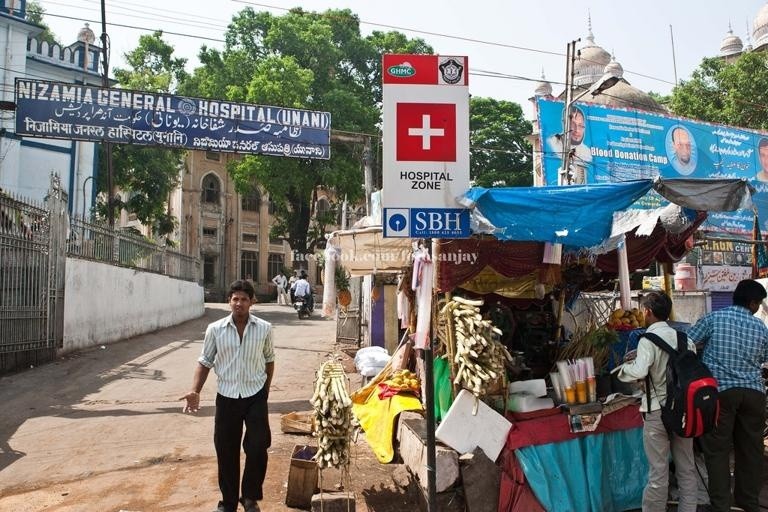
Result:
[552,376,596,406]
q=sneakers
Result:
[240,495,260,511]
[212,500,237,512]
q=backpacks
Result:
[638,330,720,438]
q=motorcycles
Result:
[294,294,315,318]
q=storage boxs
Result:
[286,444,319,507]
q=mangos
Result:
[608,308,645,328]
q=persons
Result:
[685,280,768,512]
[617,291,697,512]
[179,281,275,512]
[272,270,314,312]
[671,127,695,174]
[759,138,768,181]
[551,106,592,162]
